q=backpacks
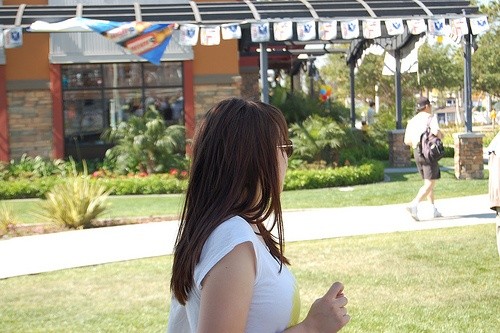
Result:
[417,114,446,166]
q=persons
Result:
[367,102,377,124]
[170,97,350,333]
[403,97,444,221]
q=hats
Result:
[416,97,430,108]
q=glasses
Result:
[276,140,293,158]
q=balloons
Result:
[320,85,331,99]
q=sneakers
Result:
[406,203,420,222]
[432,208,442,217]
